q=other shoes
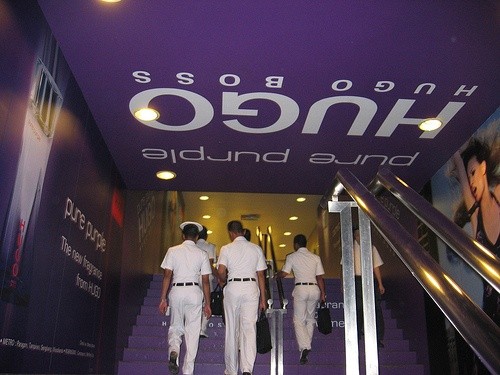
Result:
[300,348,308,365]
[200,333,209,338]
[169,350,179,375]
[378,341,384,348]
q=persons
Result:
[445,120,500,375]
[219,227,263,353]
[218,220,268,375]
[159,221,212,375]
[195,226,218,338]
[339,224,385,348]
[273,234,326,365]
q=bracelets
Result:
[160,297,166,300]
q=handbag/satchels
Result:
[318,300,332,335]
[257,309,271,354]
[210,284,223,316]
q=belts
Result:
[228,278,257,282]
[172,282,200,286]
[296,282,318,286]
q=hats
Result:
[180,222,203,233]
